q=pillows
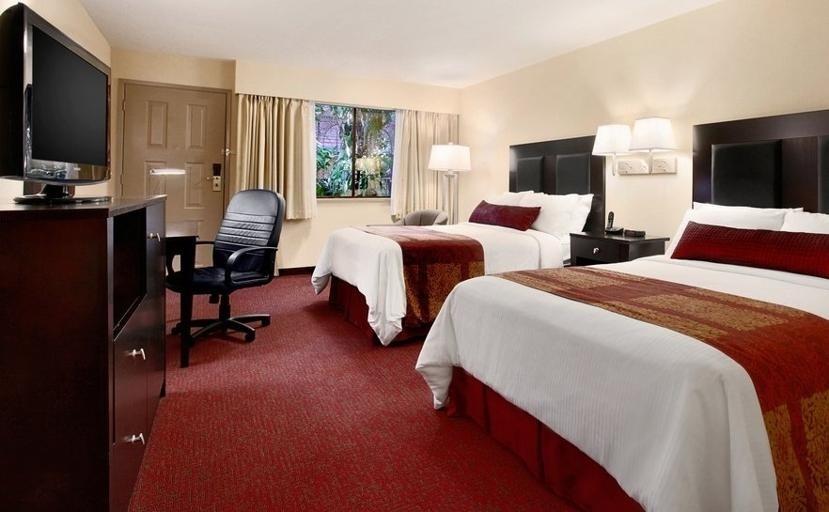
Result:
[661,202,829,279]
[469,191,594,241]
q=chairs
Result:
[165,189,286,349]
[367,209,449,225]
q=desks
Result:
[570,232,669,266]
[164,219,200,368]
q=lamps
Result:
[592,118,631,175]
[428,142,471,224]
[631,117,677,175]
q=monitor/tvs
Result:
[0,3,113,203]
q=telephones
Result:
[604,211,623,232]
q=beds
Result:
[414,110,829,511]
[311,134,605,347]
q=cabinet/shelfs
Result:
[0,194,166,510]
